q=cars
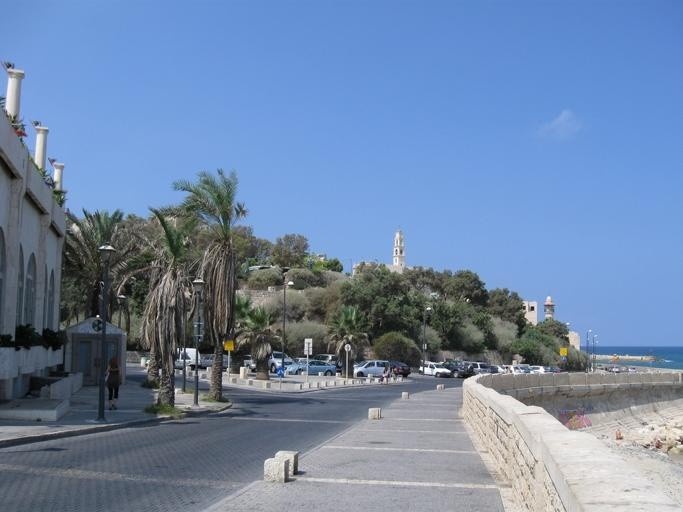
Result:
[593,363,636,373]
[353,360,411,378]
[174,347,342,376]
[418,360,560,378]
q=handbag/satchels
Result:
[104,372,109,382]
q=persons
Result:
[335,360,343,375]
[390,368,399,381]
[380,366,389,382]
[105,358,122,410]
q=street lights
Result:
[586,330,600,373]
[563,322,571,371]
[422,307,431,375]
[281,280,294,378]
[191,278,206,408]
[94,241,117,423]
